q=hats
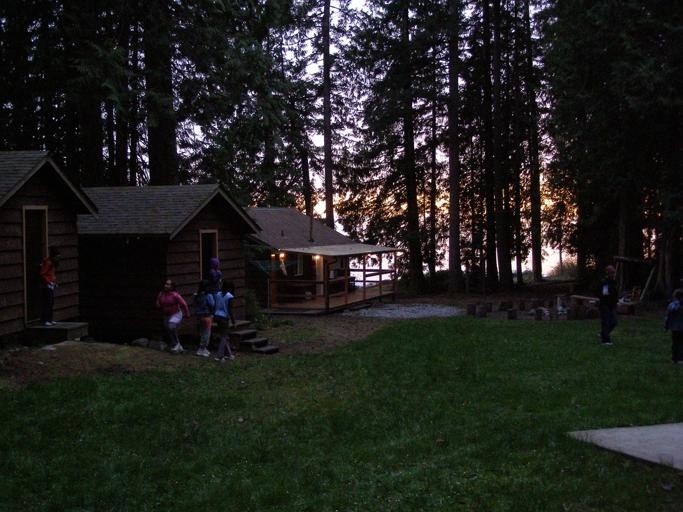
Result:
[208,258,220,265]
[48,245,59,259]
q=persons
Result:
[663,290,682,364]
[205,256,222,298]
[39,241,60,326]
[192,279,215,357]
[156,278,191,354]
[215,278,236,362]
[597,265,618,345]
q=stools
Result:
[467,298,543,320]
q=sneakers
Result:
[602,341,613,345]
[197,351,211,358]
[214,355,234,361]
[171,343,184,352]
[44,321,57,326]
